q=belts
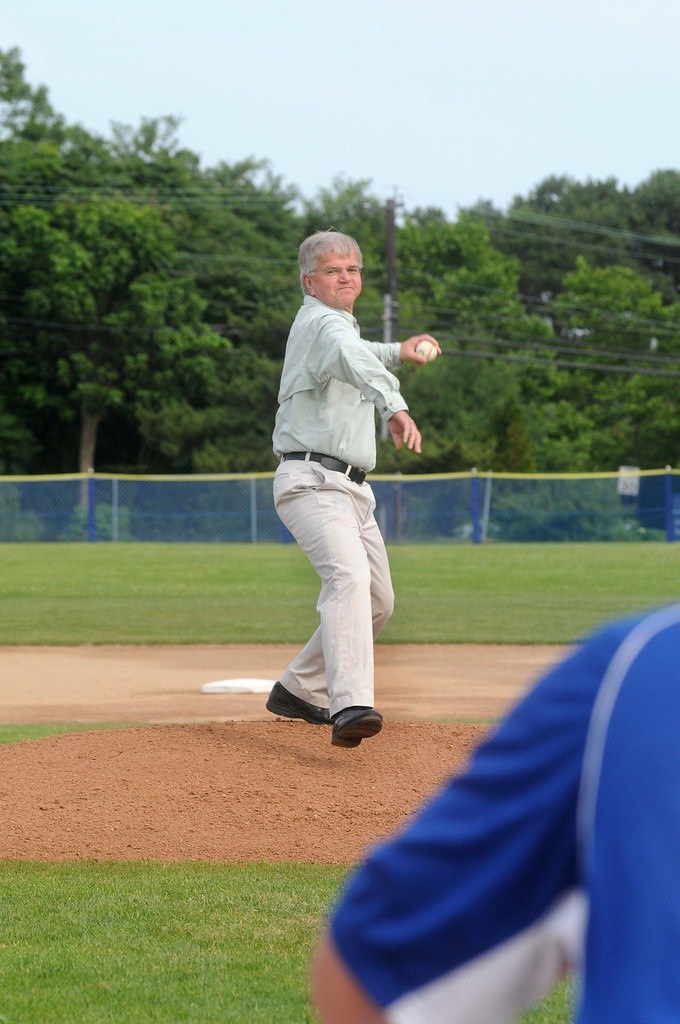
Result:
[283,452,366,484]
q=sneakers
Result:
[266,681,334,724]
[332,706,383,748]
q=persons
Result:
[265,228,443,747]
[305,599,680,1024]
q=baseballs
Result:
[415,340,437,363]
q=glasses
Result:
[309,265,362,277]
